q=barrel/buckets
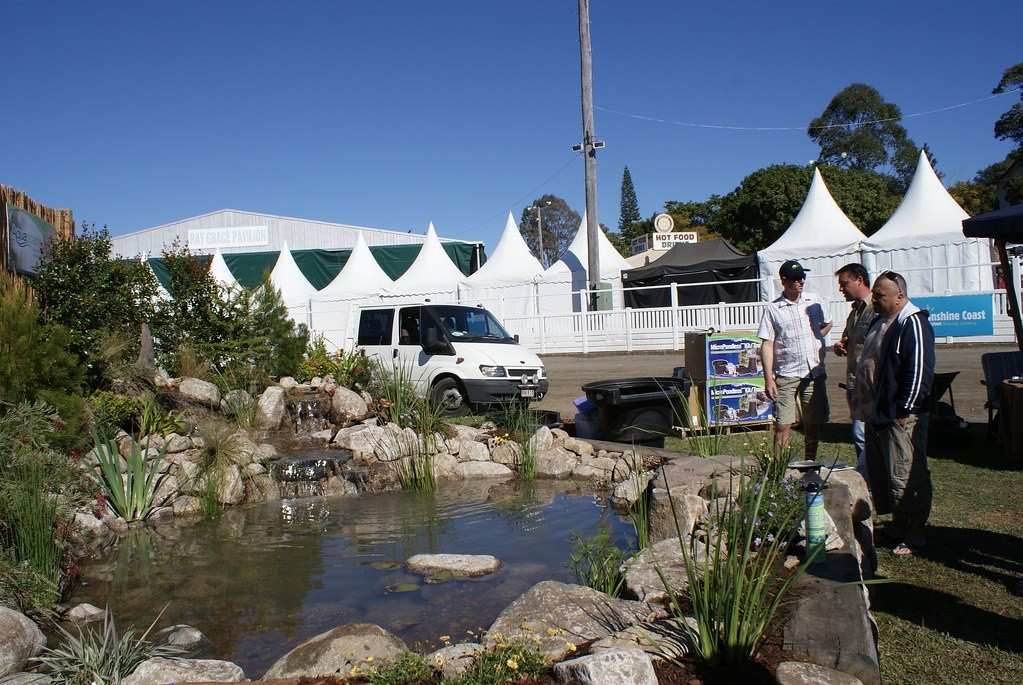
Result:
[574,412,664,448]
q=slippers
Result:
[876,531,904,544]
[889,540,928,557]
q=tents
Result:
[859,148,995,312]
[125,207,633,344]
[757,168,869,319]
[620,237,762,324]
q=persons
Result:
[849,272,936,557]
[756,259,832,465]
[834,263,877,491]
[996,274,1009,306]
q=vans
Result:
[352,299,550,418]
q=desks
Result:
[1002,379,1023,469]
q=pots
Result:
[933,370,960,402]
[787,458,846,490]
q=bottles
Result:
[739,344,757,374]
[806,482,826,564]
[740,388,757,417]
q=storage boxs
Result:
[684,327,773,426]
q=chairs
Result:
[443,317,454,329]
[930,371,959,416]
[368,319,382,330]
[404,318,419,343]
[980,351,1023,441]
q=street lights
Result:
[527,201,552,262]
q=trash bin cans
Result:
[484,410,564,432]
[582,376,692,449]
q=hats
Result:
[779,261,811,278]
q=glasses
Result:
[787,274,806,283]
[883,270,901,293]
